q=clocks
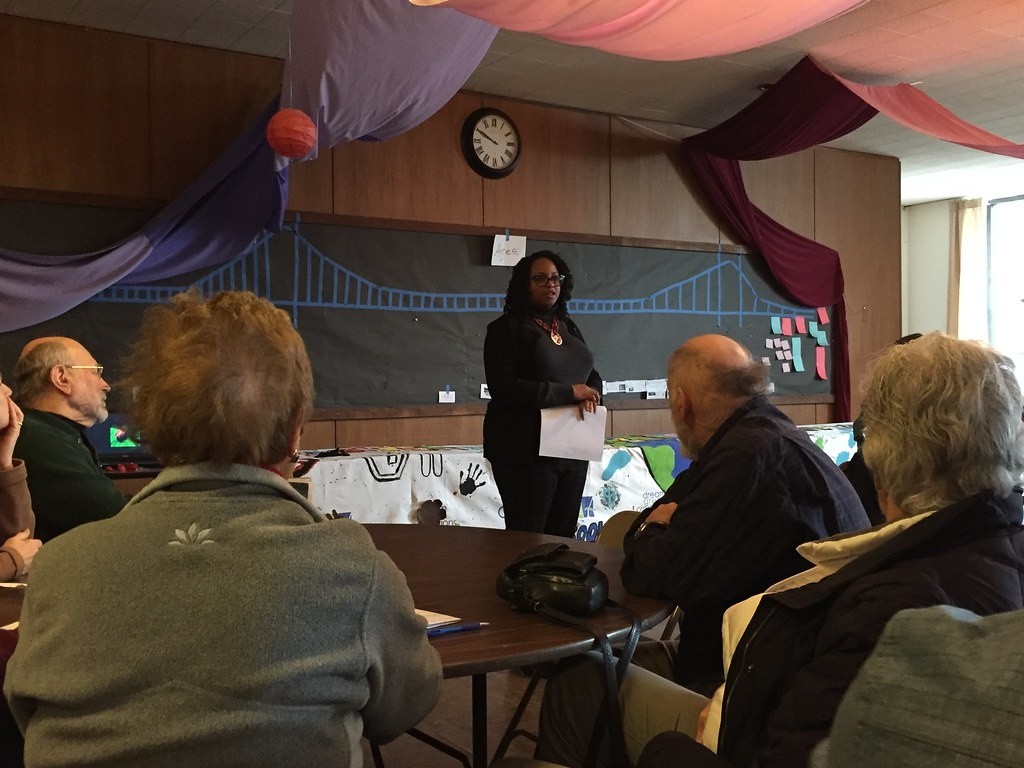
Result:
[462,106,523,180]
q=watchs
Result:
[633,521,665,538]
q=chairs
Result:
[637,485,998,768]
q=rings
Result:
[18,421,22,426]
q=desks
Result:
[298,419,861,536]
[362,521,677,766]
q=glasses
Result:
[528,274,566,287]
[61,363,103,378]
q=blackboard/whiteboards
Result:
[0,201,838,407]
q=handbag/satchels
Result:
[493,542,607,617]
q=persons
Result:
[482,250,603,679]
[13,337,133,544]
[599,334,872,699]
[530,330,1024,768]
[0,371,42,582]
[3,290,443,768]
[838,333,923,524]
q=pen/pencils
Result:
[427,621,490,637]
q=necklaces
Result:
[534,315,562,345]
